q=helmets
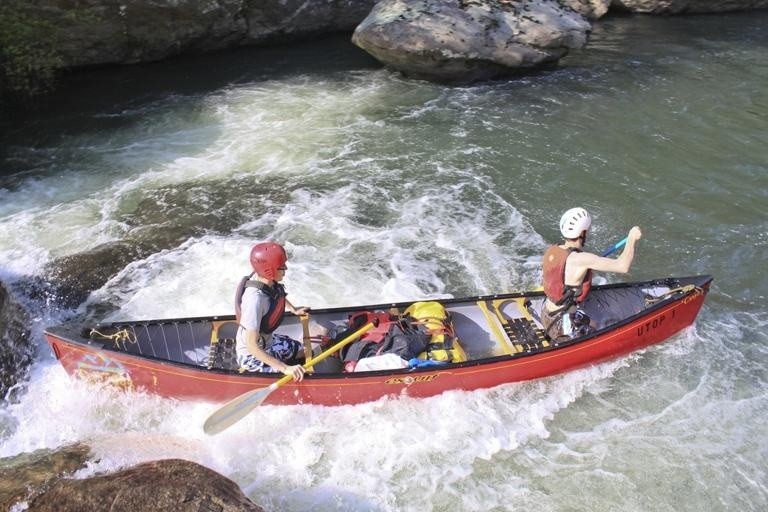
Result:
[250,243,287,281]
[560,207,592,239]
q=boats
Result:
[43,274,715,407]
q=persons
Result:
[542,207,644,342]
[235,241,311,382]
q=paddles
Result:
[204,316,379,436]
[531,238,628,292]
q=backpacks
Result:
[407,302,466,365]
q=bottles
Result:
[388,303,399,321]
